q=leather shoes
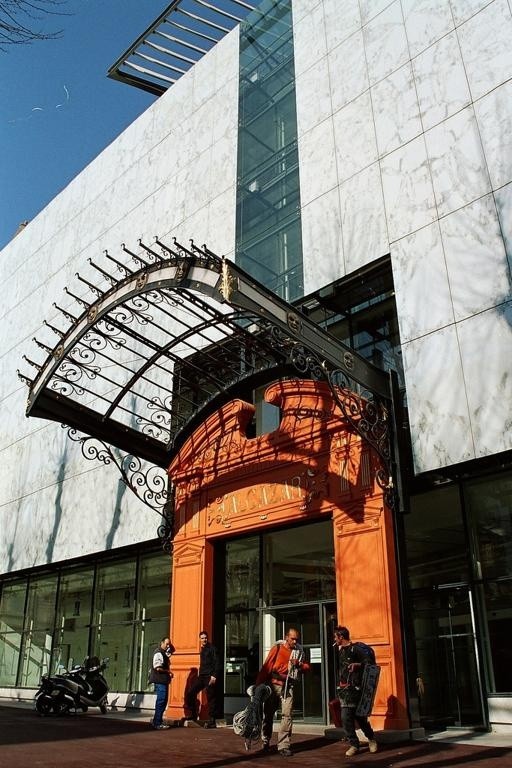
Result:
[181,716,216,729]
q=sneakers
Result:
[368,738,378,752]
[262,743,293,756]
[345,746,360,756]
[154,723,170,729]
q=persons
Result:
[181,629,221,728]
[333,624,381,756]
[255,626,311,756]
[148,635,176,729]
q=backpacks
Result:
[350,642,376,665]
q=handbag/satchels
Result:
[149,668,171,684]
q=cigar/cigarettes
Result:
[293,644,296,648]
[332,641,338,646]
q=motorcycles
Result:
[34,656,110,716]
